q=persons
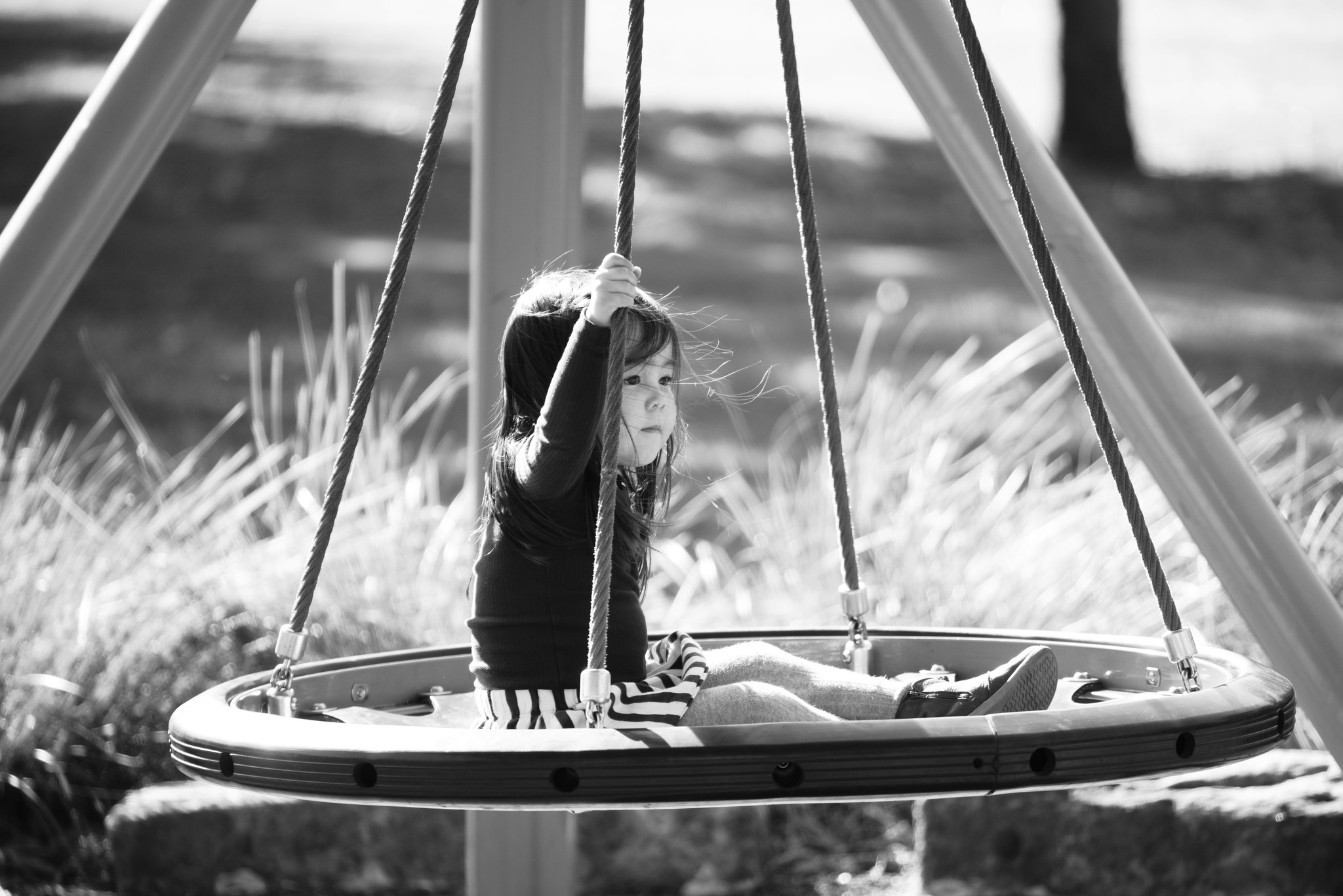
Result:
[466,251,1058,727]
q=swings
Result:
[164,0,1297,799]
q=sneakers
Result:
[894,645,1058,719]
